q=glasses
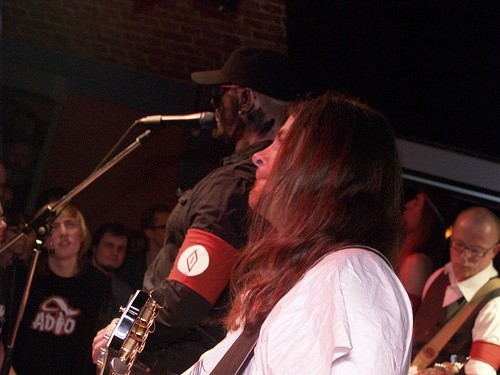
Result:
[450,235,500,258]
[151,224,166,233]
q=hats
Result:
[191,45,306,103]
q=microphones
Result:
[137,111,215,128]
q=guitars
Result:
[96,288,162,375]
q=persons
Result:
[0,141,172,375]
[176,93,413,375]
[90,46,305,375]
[390,190,500,375]
